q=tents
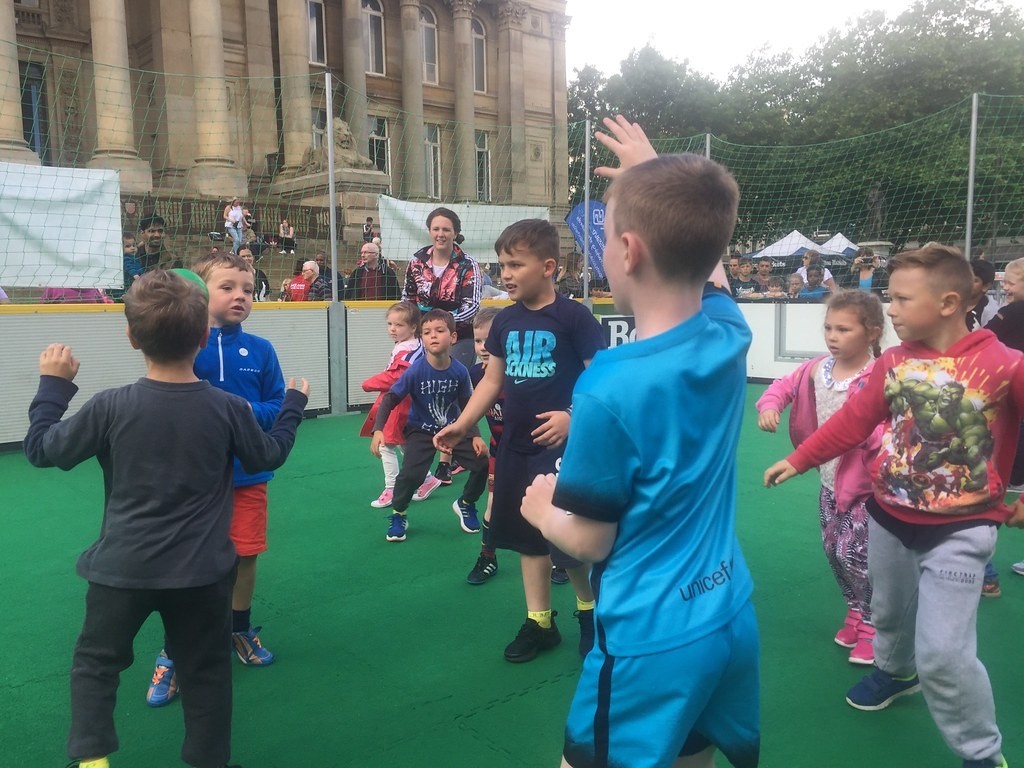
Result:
[743,230,859,273]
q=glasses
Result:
[361,250,378,255]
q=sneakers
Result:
[504,610,561,662]
[452,460,466,474]
[371,491,393,507]
[980,581,1001,596]
[578,609,595,657]
[848,621,875,665]
[147,650,178,705]
[386,510,408,541]
[834,609,862,647]
[435,462,452,483]
[232,626,274,665]
[1012,561,1023,574]
[551,567,569,583]
[467,556,498,583]
[846,669,921,709]
[452,497,480,533]
[412,475,441,501]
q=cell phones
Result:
[861,257,874,263]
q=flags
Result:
[565,200,606,279]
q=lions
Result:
[294,117,373,177]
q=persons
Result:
[764,246,1024,768]
[521,115,760,768]
[146,251,284,707]
[478,263,492,288]
[434,219,608,662]
[756,290,884,664]
[278,217,402,300]
[559,252,611,298]
[362,302,570,584]
[966,257,1024,597]
[224,197,297,302]
[41,214,182,303]
[24,270,311,768]
[401,207,481,484]
[728,247,889,300]
